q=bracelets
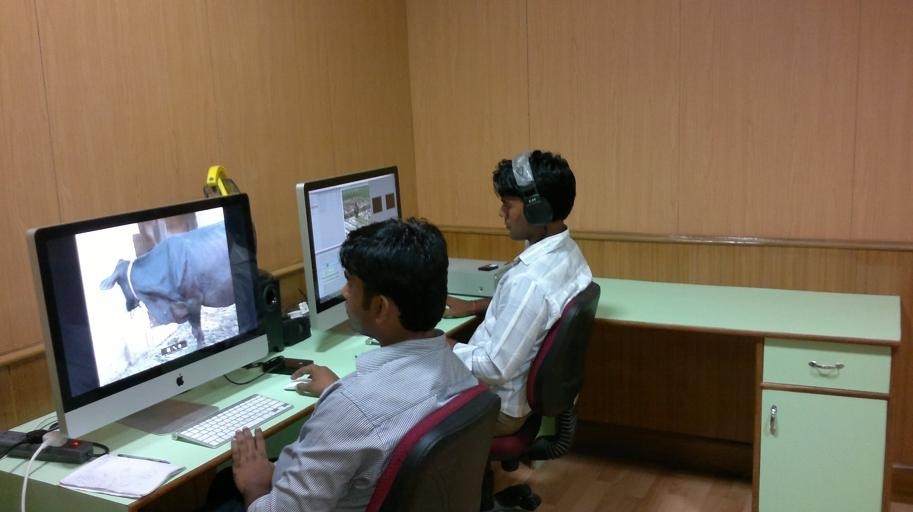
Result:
[469,300,477,313]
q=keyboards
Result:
[171,393,294,450]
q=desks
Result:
[444,258,904,512]
[0,292,487,511]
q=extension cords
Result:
[0,431,95,464]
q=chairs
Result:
[483,281,604,512]
[366,382,501,512]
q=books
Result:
[59,452,186,499]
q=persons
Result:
[354,202,359,216]
[232,217,477,511]
[439,148,591,510]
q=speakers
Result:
[252,269,284,353]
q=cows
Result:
[99,220,235,352]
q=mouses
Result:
[284,377,313,391]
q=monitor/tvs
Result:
[24,193,269,436]
[296,164,404,336]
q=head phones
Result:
[512,153,552,225]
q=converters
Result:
[264,357,313,375]
